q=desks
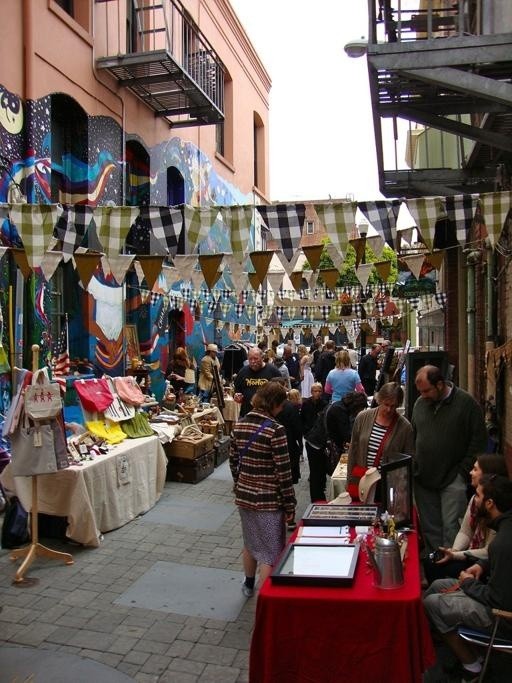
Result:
[247,496,441,683]
[210,393,241,423]
[0,428,170,548]
[148,401,225,444]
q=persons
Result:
[428,451,508,580]
[343,382,413,515]
[423,474,512,683]
[409,365,485,589]
[226,380,298,598]
[163,335,401,502]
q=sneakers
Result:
[241,576,254,598]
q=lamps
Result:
[341,33,371,59]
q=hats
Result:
[207,343,218,352]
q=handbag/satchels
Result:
[347,465,367,499]
[327,448,345,476]
[74,376,154,444]
[3,369,69,476]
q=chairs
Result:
[458,605,511,682]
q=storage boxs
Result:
[167,448,217,484]
[166,432,215,459]
[215,436,232,466]
